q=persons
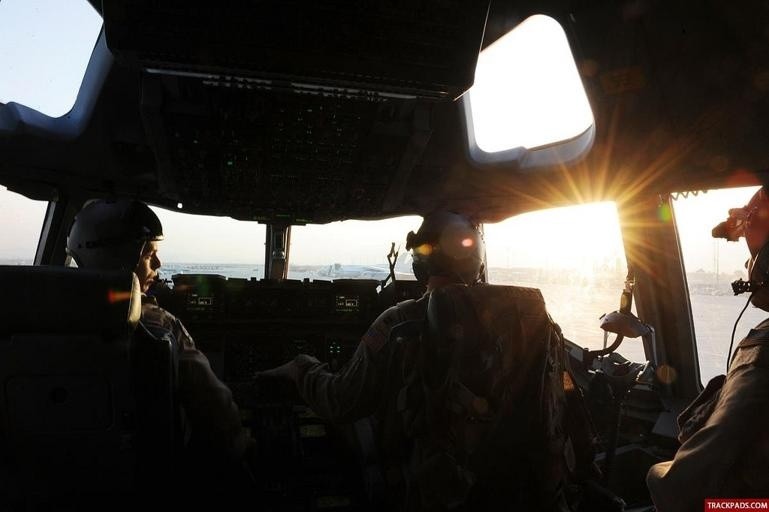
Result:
[254,208,481,469]
[61,194,249,456]
[646,185,769,511]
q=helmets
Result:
[713,184,769,311]
[407,211,486,284]
[65,199,165,273]
